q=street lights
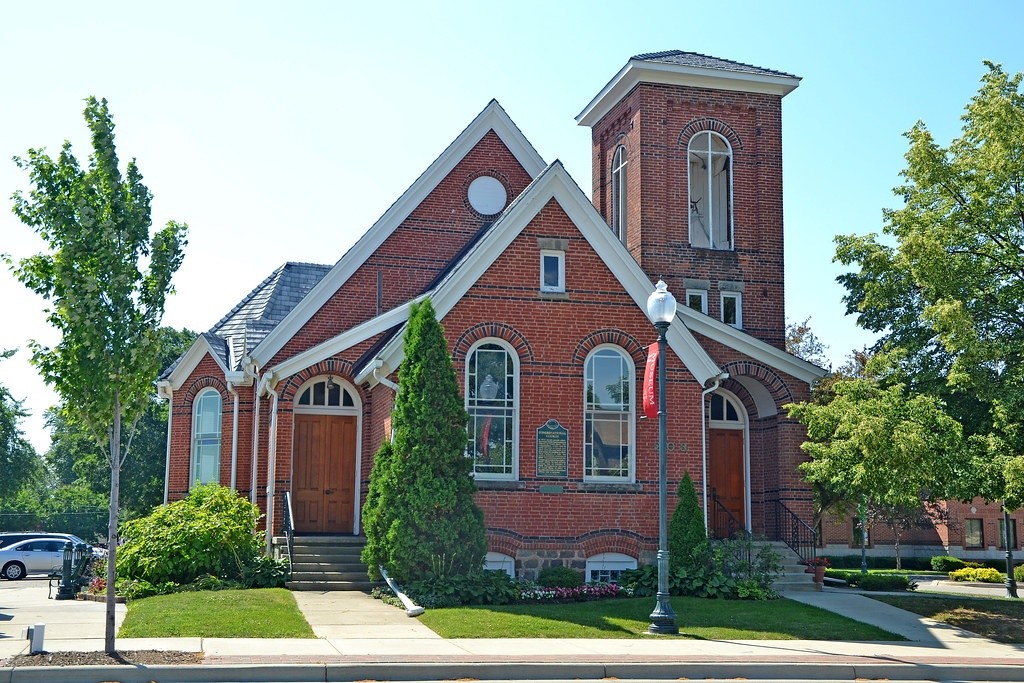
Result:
[646,275,678,634]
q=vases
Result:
[805,566,825,582]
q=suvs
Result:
[0,531,108,560]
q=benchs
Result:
[46,555,86,599]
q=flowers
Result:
[797,556,831,569]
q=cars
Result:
[0,537,109,581]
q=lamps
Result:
[61,541,94,565]
[326,374,333,389]
